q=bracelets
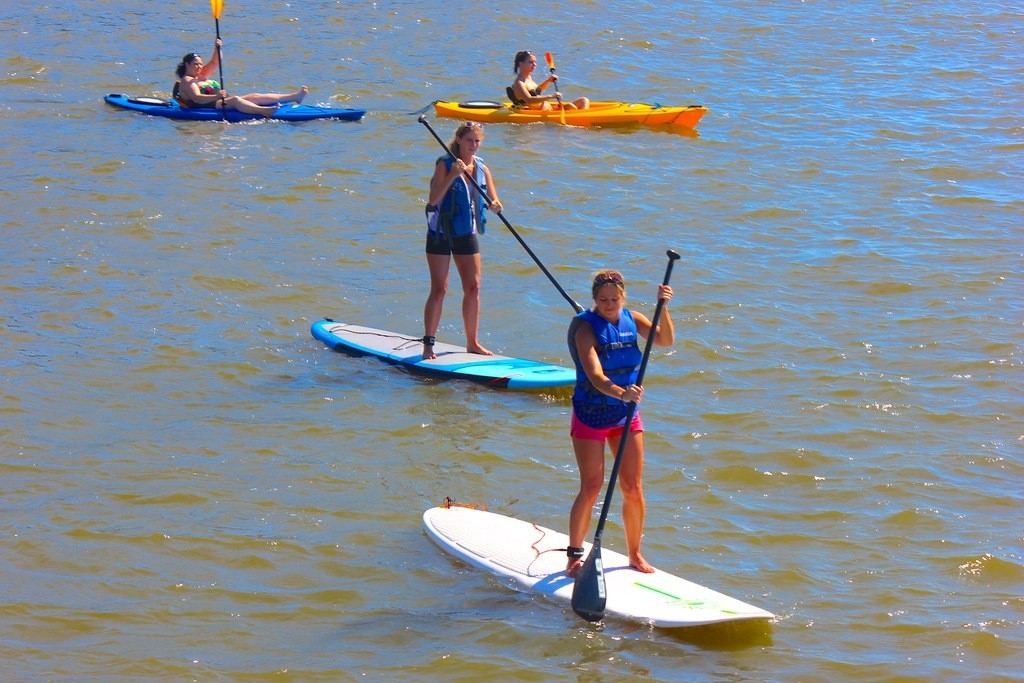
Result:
[621,392,626,401]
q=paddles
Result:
[418,114,585,315]
[209,0,227,122]
[571,249,682,622]
[545,51,566,125]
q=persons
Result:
[422,120,502,360]
[512,50,589,112]
[175,53,308,119]
[567,270,674,579]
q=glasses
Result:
[465,121,484,129]
[594,273,622,285]
[191,53,200,57]
[524,51,534,55]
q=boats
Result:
[434,98,711,133]
[103,89,368,126]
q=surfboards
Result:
[311,317,576,389]
[423,505,775,628]
